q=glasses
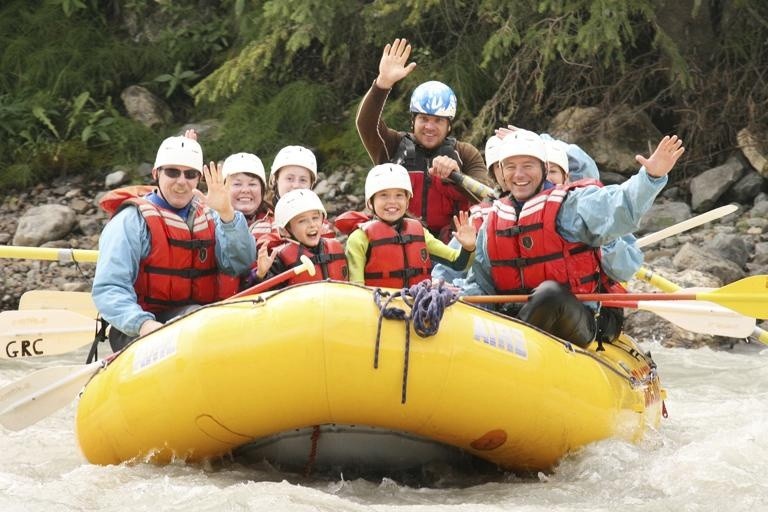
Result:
[158,166,201,180]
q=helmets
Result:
[152,135,204,178]
[270,144,318,188]
[221,151,267,190]
[409,80,458,121]
[273,188,328,232]
[484,129,569,180]
[363,162,414,211]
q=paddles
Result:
[0,309,117,359]
[636,205,739,247]
[459,275,767,323]
[585,295,757,338]
[0,256,316,431]
[635,264,768,348]
[17,288,107,326]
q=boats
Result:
[77,281,667,489]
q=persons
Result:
[243,187,349,293]
[265,145,317,207]
[355,38,488,245]
[344,163,477,290]
[91,135,258,354]
[185,129,287,287]
